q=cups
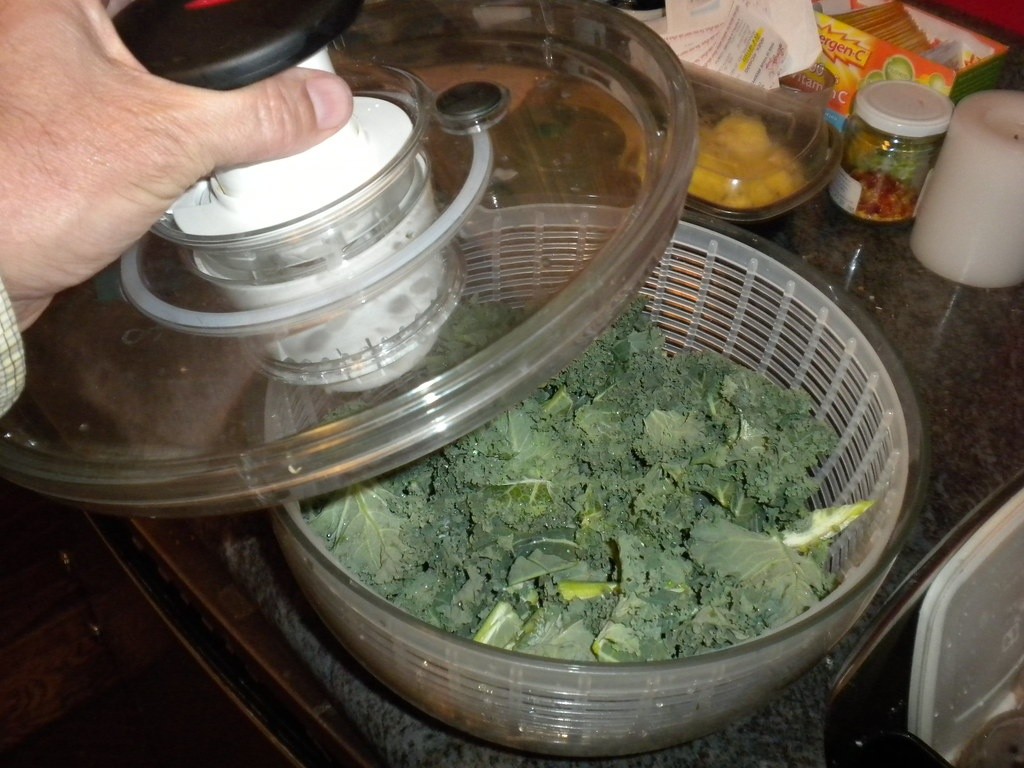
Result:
[776,63,836,116]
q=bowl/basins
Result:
[259,195,932,758]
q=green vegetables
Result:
[287,285,876,668]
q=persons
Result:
[0,1,359,423]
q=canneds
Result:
[829,79,951,226]
